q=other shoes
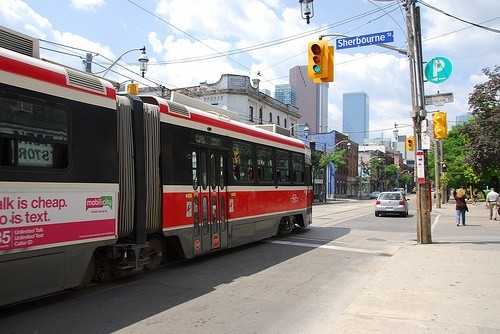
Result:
[490,218,492,220]
[494,218,497,220]
[457,223,459,226]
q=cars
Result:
[375,192,409,217]
[411,188,416,194]
[371,191,380,199]
[434,190,442,199]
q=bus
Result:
[1,26,313,311]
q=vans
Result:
[394,188,405,197]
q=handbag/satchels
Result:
[465,204,469,212]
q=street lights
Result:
[324,140,352,203]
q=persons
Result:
[473,189,479,202]
[453,190,456,198]
[486,188,499,220]
[455,189,467,226]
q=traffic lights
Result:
[442,162,447,172]
[308,39,329,78]
[407,138,415,152]
[234,151,240,165]
